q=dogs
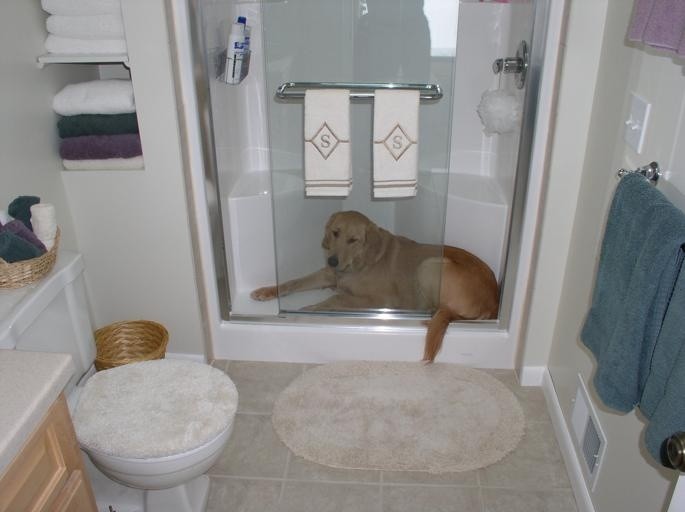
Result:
[250,210,500,367]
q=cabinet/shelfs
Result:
[0,346,99,511]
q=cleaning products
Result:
[224,15,251,87]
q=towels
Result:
[301,86,354,199]
[35,0,144,172]
[577,167,683,415]
[371,88,422,200]
[0,195,58,263]
[626,0,685,60]
[639,254,684,469]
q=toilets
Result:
[1,253,239,512]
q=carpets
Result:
[268,354,529,476]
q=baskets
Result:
[1,225,61,290]
